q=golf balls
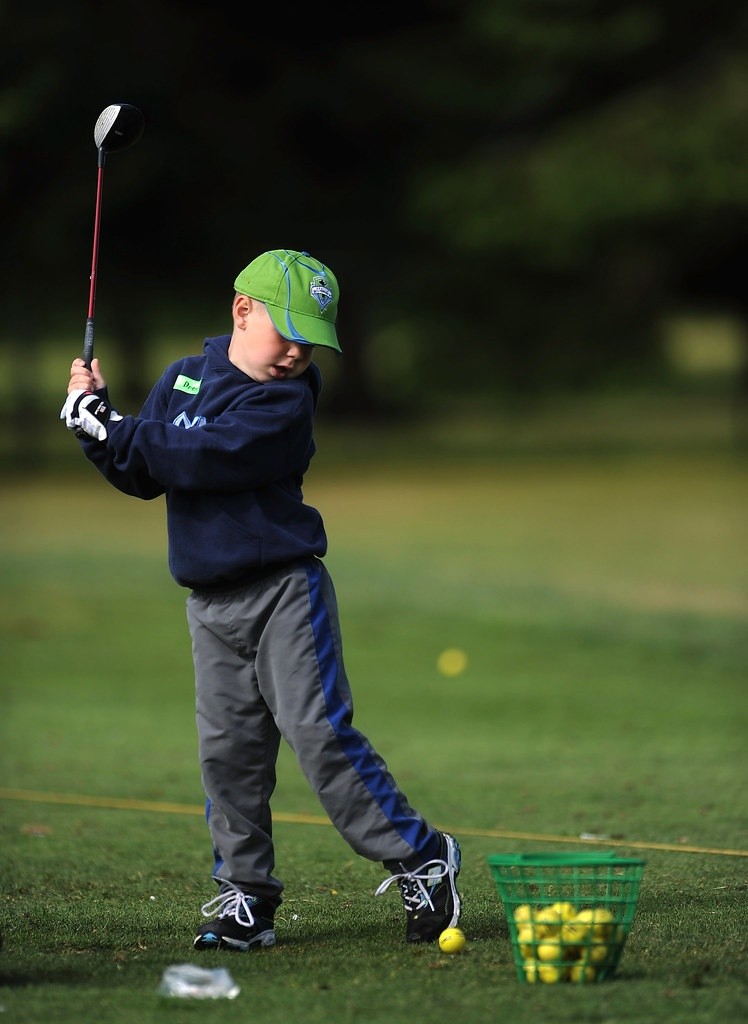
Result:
[437,648,468,677]
[514,902,625,985]
[439,927,466,953]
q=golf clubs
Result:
[75,102,146,440]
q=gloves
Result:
[60,389,122,441]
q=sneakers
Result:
[192,874,277,952]
[374,830,461,945]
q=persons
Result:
[59,249,461,950]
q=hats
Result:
[234,249,343,357]
